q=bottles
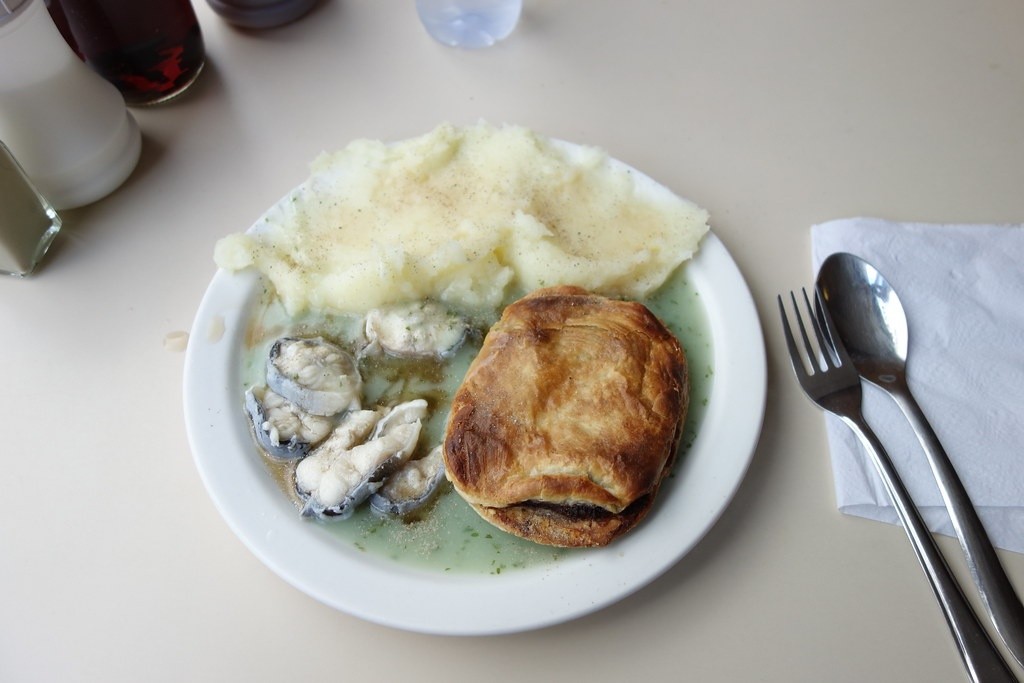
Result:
[0,0,144,211]
[205,1,323,30]
[0,137,63,276]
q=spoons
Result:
[812,251,1024,668]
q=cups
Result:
[41,1,208,109]
[416,1,522,51]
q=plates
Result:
[181,136,767,637]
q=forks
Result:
[777,283,1019,683]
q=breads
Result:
[443,283,691,548]
